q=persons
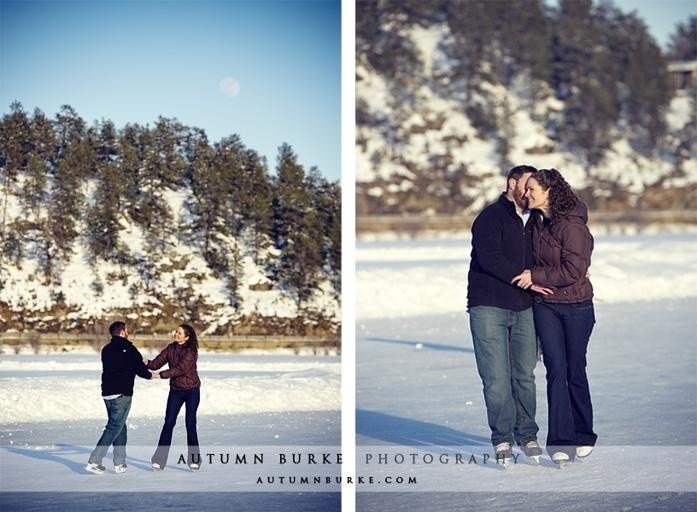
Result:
[510,168,598,469]
[466,165,592,470]
[141,324,202,472]
[83,320,157,474]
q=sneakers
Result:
[518,439,544,457]
[87,461,106,472]
[494,441,513,461]
[575,446,594,457]
[189,462,200,469]
[551,451,571,461]
[113,462,128,468]
[152,462,162,469]
